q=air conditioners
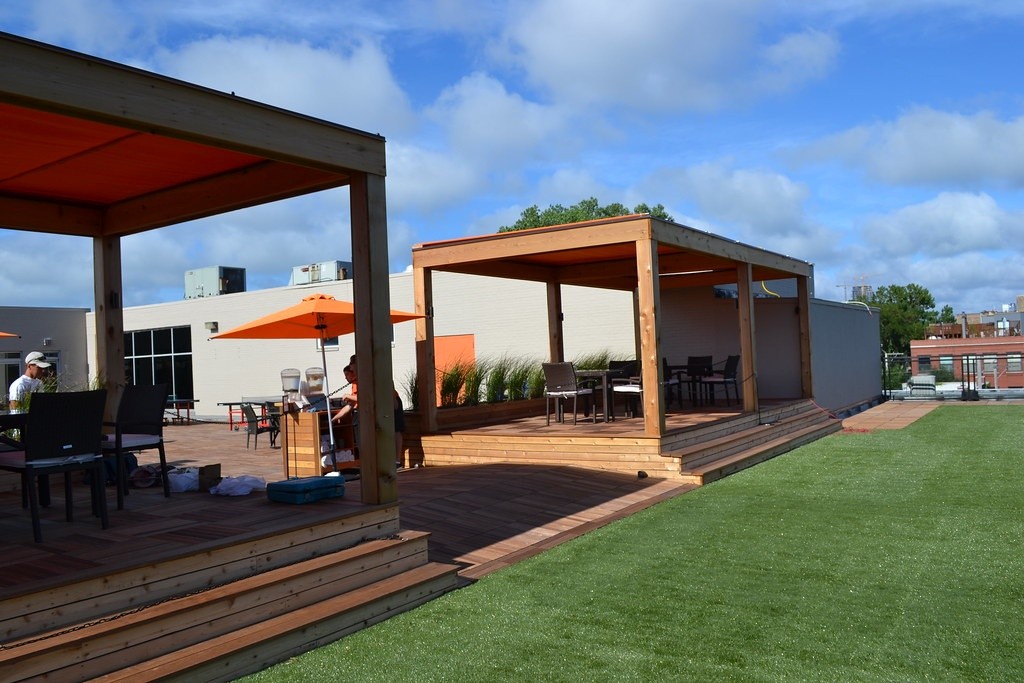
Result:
[204,321,218,330]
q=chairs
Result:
[661,357,684,411]
[594,359,642,418]
[0,389,110,544]
[97,384,171,510]
[541,362,597,426]
[702,355,741,411]
[677,355,714,409]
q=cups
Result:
[281,369,301,393]
[305,367,325,395]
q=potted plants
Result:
[402,352,615,434]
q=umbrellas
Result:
[207,290,428,453]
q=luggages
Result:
[267,474,345,505]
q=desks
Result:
[668,363,690,411]
[1,409,29,452]
[166,399,200,424]
[217,401,268,431]
[558,369,625,424]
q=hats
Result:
[25,351,51,368]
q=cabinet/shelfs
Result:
[273,396,354,480]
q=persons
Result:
[8,351,52,442]
[340,353,370,452]
[329,363,406,469]
